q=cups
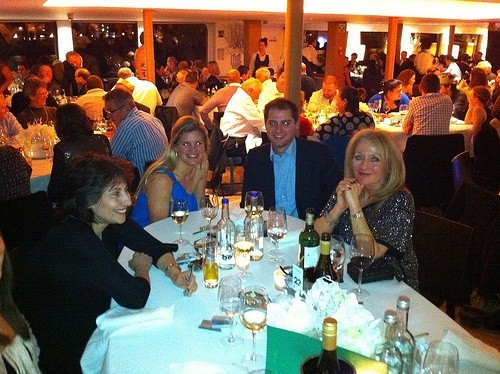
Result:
[422,339,460,374]
[244,191,264,216]
[201,237,218,289]
[329,234,346,272]
[300,355,356,374]
[67,96,78,103]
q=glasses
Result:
[103,104,129,115]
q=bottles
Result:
[375,310,403,374]
[207,81,226,100]
[316,317,341,374]
[243,192,264,261]
[298,208,320,280]
[50,89,66,105]
[388,295,416,374]
[216,198,236,270]
[313,232,338,284]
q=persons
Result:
[0,37,500,188]
[241,98,342,221]
[0,231,41,374]
[130,116,210,227]
[313,129,419,292]
[35,152,197,374]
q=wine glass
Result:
[349,233,375,299]
[171,199,190,246]
[266,206,287,268]
[367,100,407,127]
[218,275,244,347]
[234,225,255,280]
[200,194,218,242]
[238,284,269,367]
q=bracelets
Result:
[349,211,363,219]
[322,210,340,225]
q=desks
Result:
[262,117,472,157]
[81,210,499,374]
[20,139,60,208]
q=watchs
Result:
[164,264,180,276]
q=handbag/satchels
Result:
[346,258,395,284]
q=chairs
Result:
[0,190,52,251]
[155,105,180,144]
[403,134,500,320]
[206,123,244,196]
